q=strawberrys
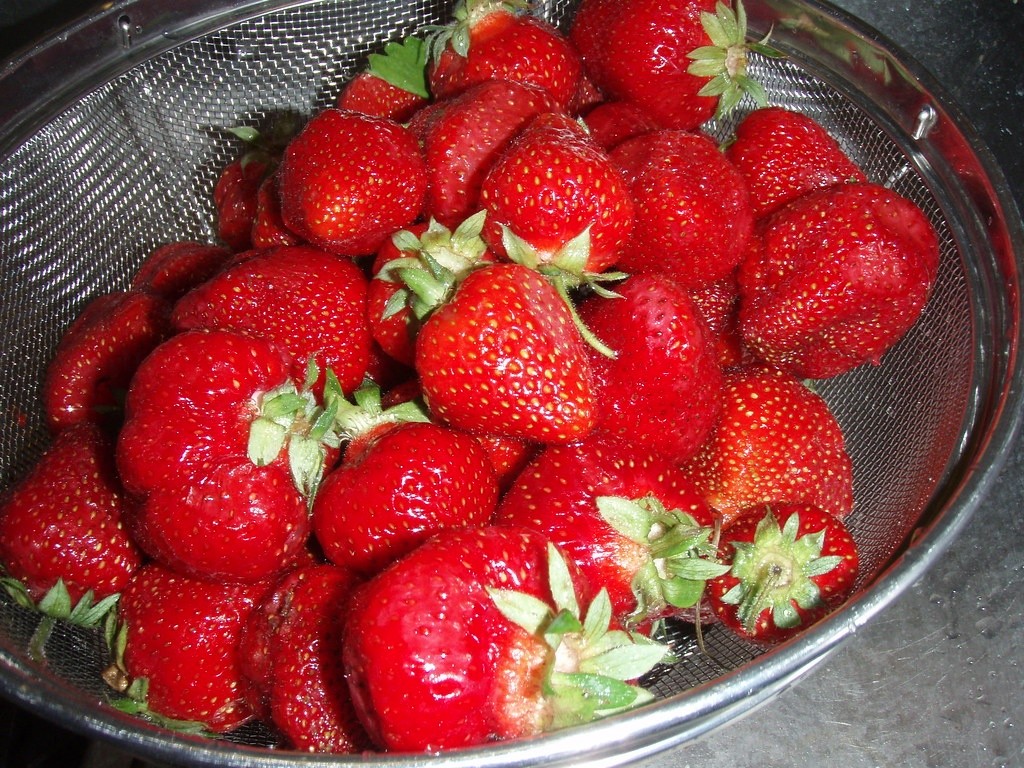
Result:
[0,0,939,757]
